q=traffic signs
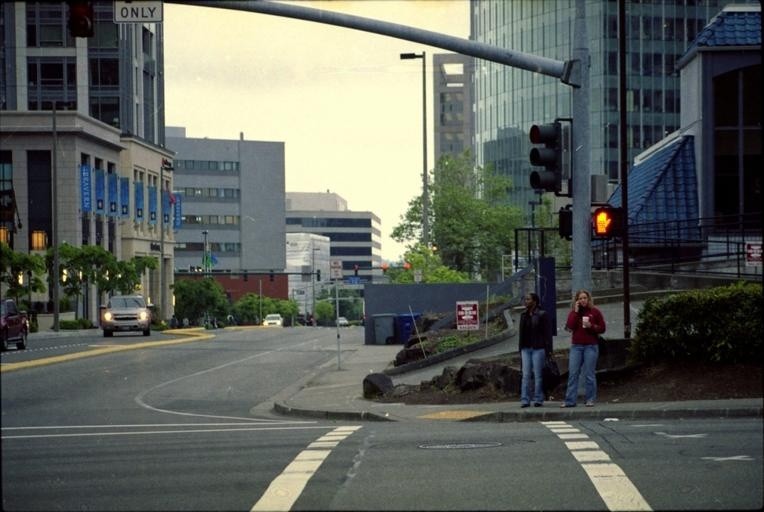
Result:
[115,0,163,22]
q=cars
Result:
[101,296,155,337]
[335,316,348,326]
[263,314,284,327]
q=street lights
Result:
[50,96,74,329]
[312,247,320,327]
[398,49,430,250]
[201,230,210,327]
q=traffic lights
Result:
[383,264,386,274]
[525,123,561,194]
[354,264,358,276]
[67,0,93,36]
[405,263,409,271]
[592,207,624,239]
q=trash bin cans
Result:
[371,312,421,345]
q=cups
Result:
[582,316,589,329]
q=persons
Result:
[517,293,552,407]
[559,290,605,407]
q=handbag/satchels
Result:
[546,353,561,376]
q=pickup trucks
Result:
[0,298,30,349]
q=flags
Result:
[167,184,176,207]
[201,249,219,268]
